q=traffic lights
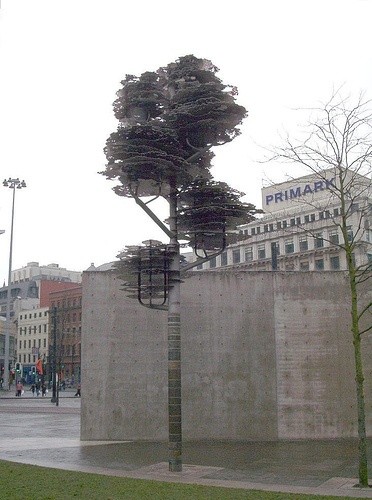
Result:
[31,366,36,375]
[15,362,21,373]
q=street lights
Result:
[2,177,28,391]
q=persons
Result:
[16,370,75,396]
[74,380,81,396]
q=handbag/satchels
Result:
[21,390,24,396]
[16,393,18,396]
[46,390,49,394]
